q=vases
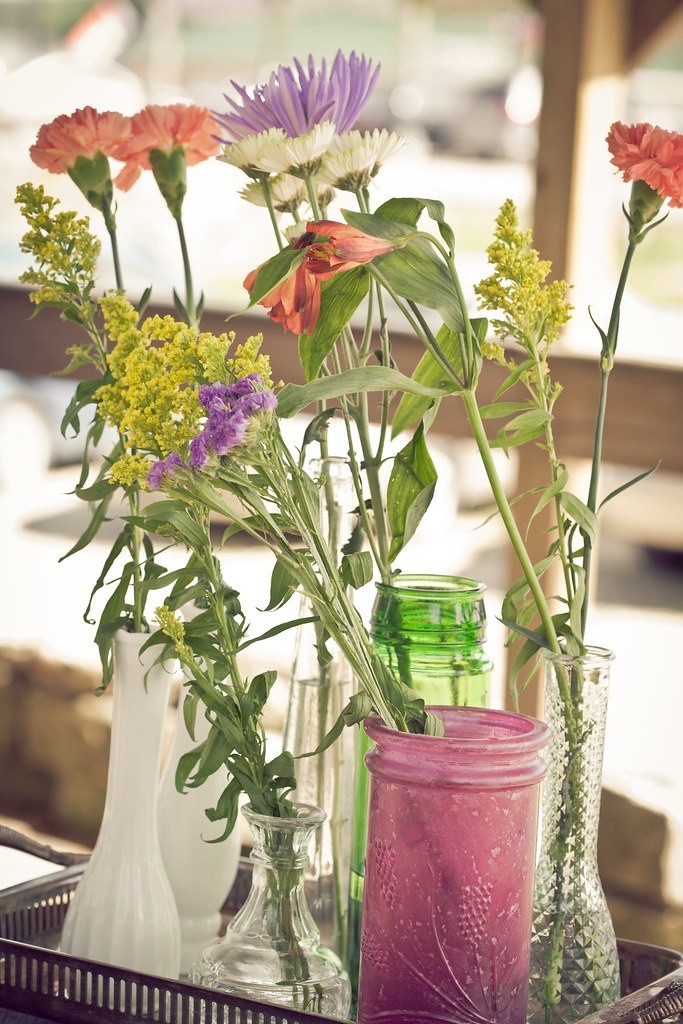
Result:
[349,574,494,1024]
[357,705,552,1024]
[526,645,625,1024]
[187,801,351,1024]
[282,585,356,946]
[54,625,180,1016]
[156,599,241,976]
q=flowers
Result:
[15,48,683,1024]
